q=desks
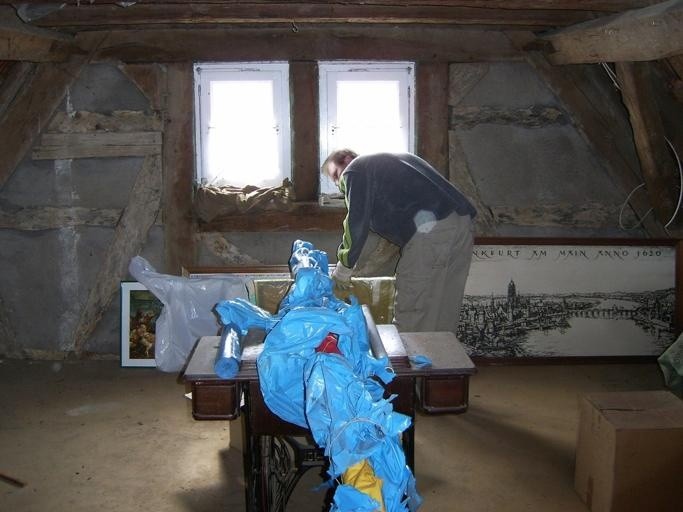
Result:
[177,329,476,512]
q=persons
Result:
[320,149,477,333]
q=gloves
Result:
[331,259,358,292]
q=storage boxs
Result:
[568,387,680,512]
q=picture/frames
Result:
[118,278,164,371]
